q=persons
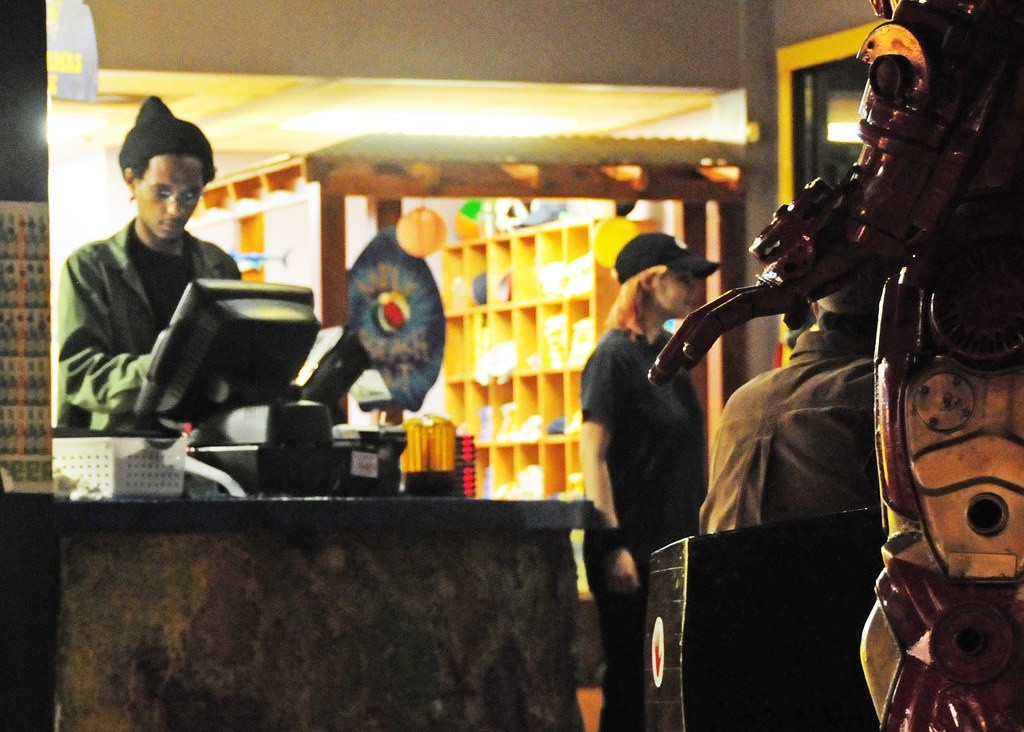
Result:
[583,232,724,732]
[57,96,243,433]
[698,275,880,535]
[649,0,1024,732]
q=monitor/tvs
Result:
[134,278,319,423]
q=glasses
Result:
[142,183,202,206]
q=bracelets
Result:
[603,527,634,550]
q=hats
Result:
[818,280,881,314]
[616,233,721,285]
[119,96,215,187]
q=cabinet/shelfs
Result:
[442,219,662,500]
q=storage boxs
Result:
[49,429,184,500]
